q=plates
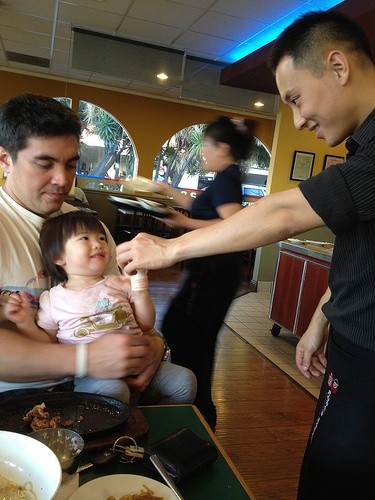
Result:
[65,474,180,500]
[135,196,164,210]
[0,391,131,440]
[108,196,140,206]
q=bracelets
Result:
[73,343,91,379]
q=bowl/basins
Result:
[25,428,86,481]
[0,430,64,500]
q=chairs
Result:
[123,208,159,244]
[152,206,190,270]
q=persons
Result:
[3,210,198,405]
[0,92,170,407]
[116,10,375,500]
[127,113,258,437]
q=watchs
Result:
[148,333,170,363]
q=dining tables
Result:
[79,403,257,500]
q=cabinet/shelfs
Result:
[268,238,335,340]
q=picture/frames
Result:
[323,154,345,172]
[290,149,316,181]
